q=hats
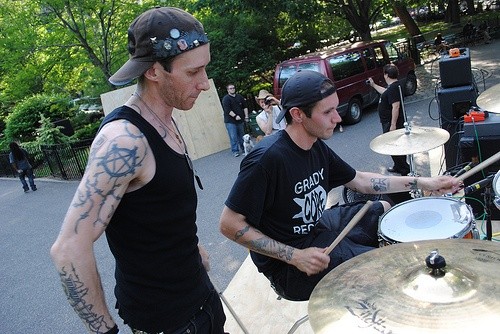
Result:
[275,69,336,124]
[255,90,273,103]
[108,6,209,86]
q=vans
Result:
[273,39,418,125]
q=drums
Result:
[377,198,481,247]
[492,168,500,211]
[309,240,499,331]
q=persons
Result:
[432,32,454,55]
[216,70,464,302]
[255,90,287,136]
[463,19,478,43]
[479,20,494,44]
[216,82,253,157]
[7,136,38,192]
[365,63,414,178]
[49,3,228,334]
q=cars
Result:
[74,96,104,123]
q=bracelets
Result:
[233,114,238,118]
[244,116,249,119]
[96,323,122,334]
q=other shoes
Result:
[232,152,239,157]
[32,186,37,191]
[24,187,29,192]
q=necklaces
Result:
[138,98,205,191]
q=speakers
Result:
[460,137,500,221]
[439,48,472,88]
[436,74,475,169]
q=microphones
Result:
[465,175,494,194]
[452,162,472,177]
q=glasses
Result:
[229,88,235,90]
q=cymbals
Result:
[475,83,499,115]
[369,126,451,156]
